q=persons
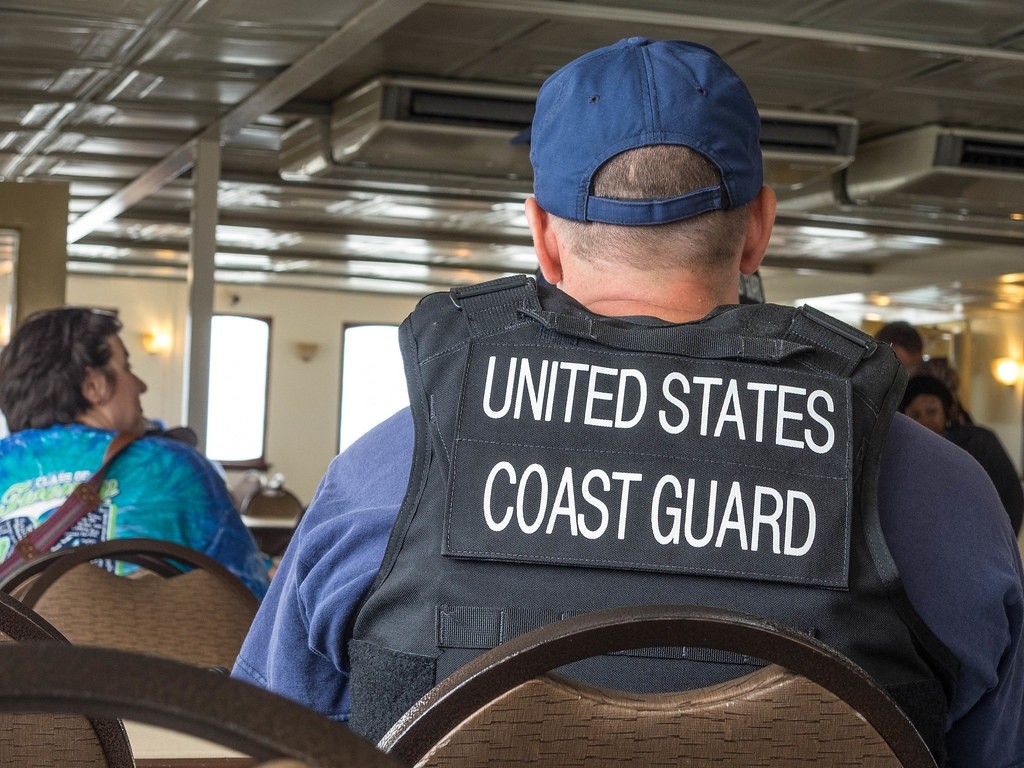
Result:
[222,34,1024,767]
[871,320,1024,539]
[0,307,275,607]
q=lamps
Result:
[291,341,320,361]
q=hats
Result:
[509,34,763,220]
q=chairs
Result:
[233,470,305,528]
[0,537,941,768]
